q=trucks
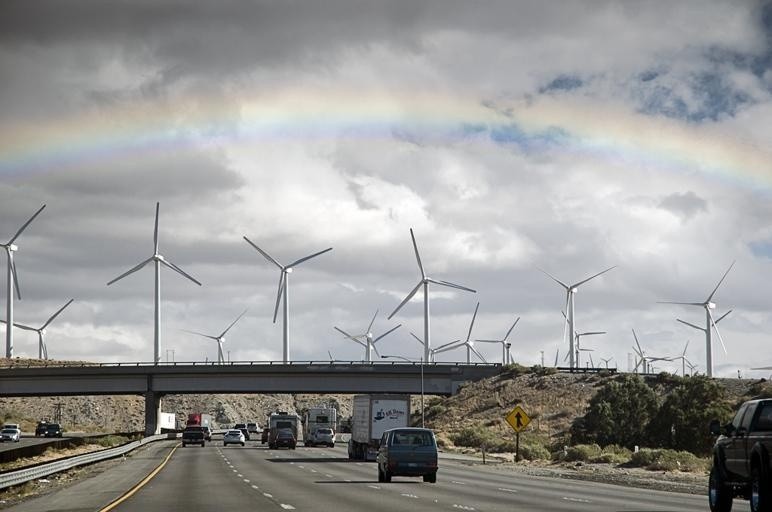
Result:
[185,414,211,428]
[348,394,410,461]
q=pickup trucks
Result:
[709,398,772,512]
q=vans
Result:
[376,427,438,483]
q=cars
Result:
[223,422,269,446]
[35,420,63,438]
[314,428,335,448]
[0,424,21,443]
[275,431,296,450]
[181,425,211,447]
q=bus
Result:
[267,412,298,447]
[301,407,336,446]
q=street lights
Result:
[381,355,425,427]
